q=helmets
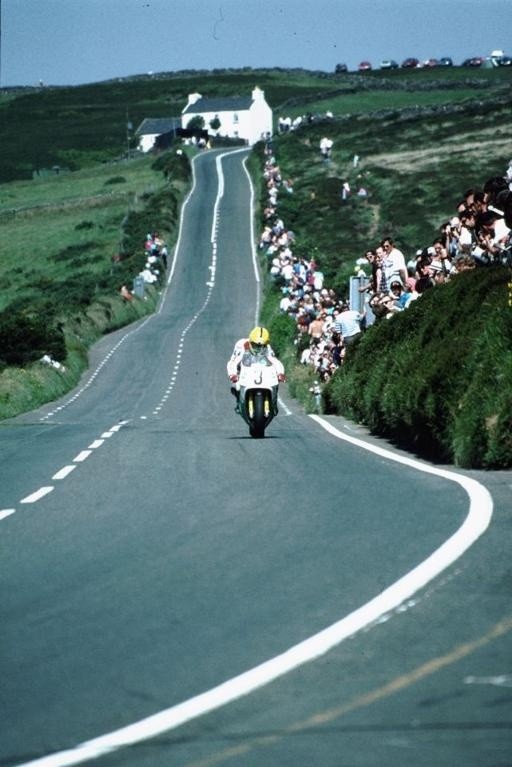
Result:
[248,327,270,356]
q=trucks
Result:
[486,50,512,65]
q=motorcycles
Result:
[234,349,286,438]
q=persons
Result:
[261,133,367,395]
[183,138,189,144]
[207,137,214,150]
[224,327,286,437]
[356,180,511,331]
[199,135,206,147]
[191,135,197,145]
[119,230,169,305]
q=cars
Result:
[359,60,371,70]
[335,63,347,72]
[440,57,452,67]
[424,59,438,67]
[470,57,485,66]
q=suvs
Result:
[380,60,398,69]
[401,58,418,67]
